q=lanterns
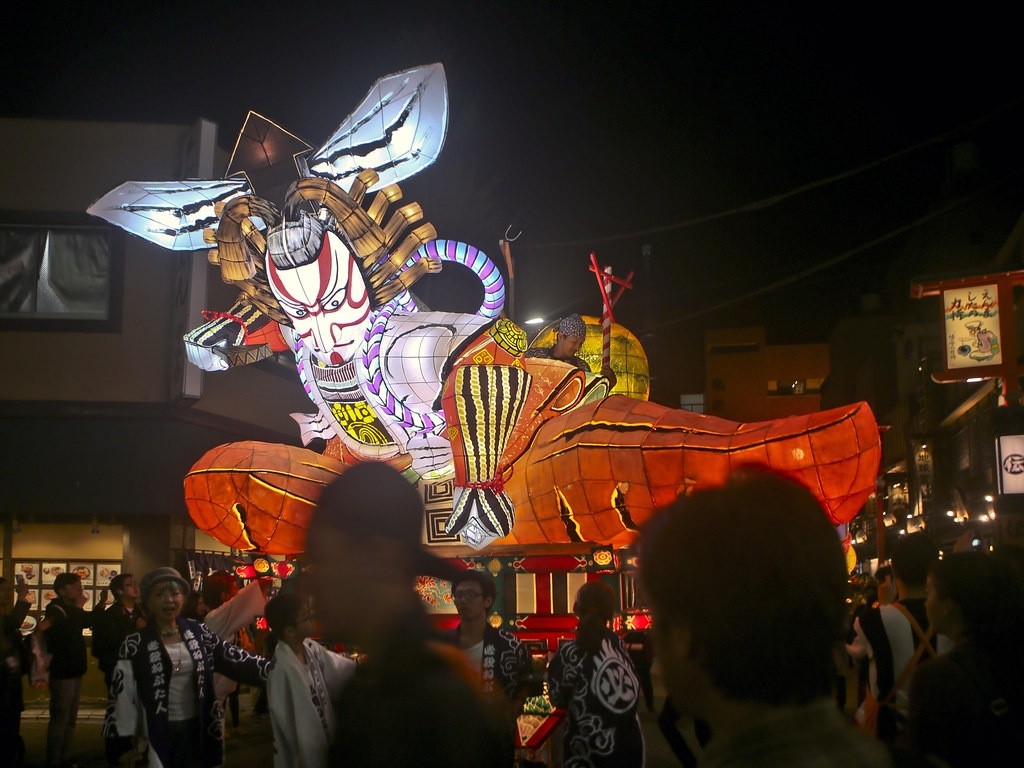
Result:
[87,63,882,556]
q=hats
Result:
[317,459,467,581]
[139,567,191,603]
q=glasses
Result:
[451,592,484,602]
[122,582,138,587]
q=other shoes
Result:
[233,726,248,735]
[223,728,232,738]
[63,759,79,768]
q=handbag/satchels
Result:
[851,695,879,739]
[28,633,48,689]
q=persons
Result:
[101,568,272,768]
[908,551,1024,768]
[97,573,145,768]
[552,583,646,768]
[187,571,274,735]
[265,595,368,768]
[846,530,1024,744]
[525,313,617,392]
[43,572,107,768]
[636,472,892,768]
[0,577,53,768]
[307,460,514,768]
[450,570,530,717]
